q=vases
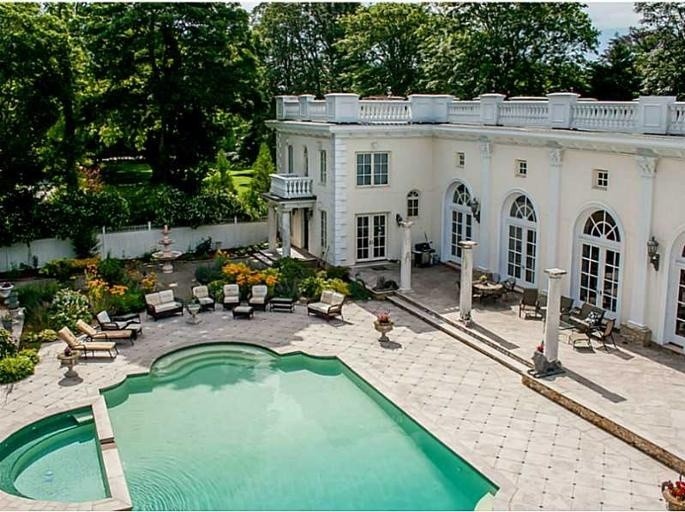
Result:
[662,488,685,511]
[58,350,79,378]
[187,304,201,324]
[373,321,392,343]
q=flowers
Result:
[64,346,71,356]
[662,479,685,499]
[377,311,390,322]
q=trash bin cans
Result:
[415,242,432,268]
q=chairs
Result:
[411,242,435,267]
[191,286,216,311]
[246,284,268,312]
[58,325,119,358]
[223,284,240,310]
[96,309,142,339]
[456,270,617,351]
[75,318,136,345]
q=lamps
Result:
[647,235,660,271]
[470,197,480,223]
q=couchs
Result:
[307,290,346,322]
[144,289,184,322]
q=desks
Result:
[269,296,294,313]
